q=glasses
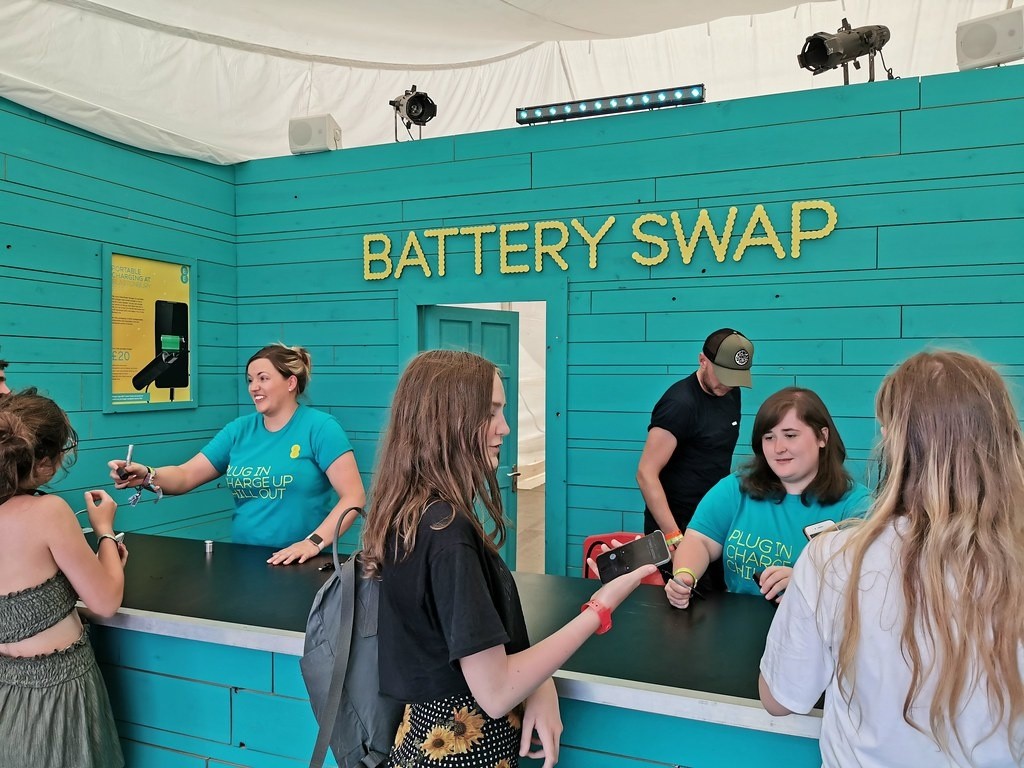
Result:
[60,438,77,456]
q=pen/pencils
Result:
[661,569,708,601]
[123,445,134,469]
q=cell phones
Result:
[752,572,780,609]
[594,530,671,585]
[804,519,841,541]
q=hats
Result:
[703,328,755,389]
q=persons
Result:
[0,386,127,768]
[360,349,657,768]
[759,345,1024,766]
[636,327,755,590]
[0,359,12,395]
[664,385,875,608]
[108,344,367,565]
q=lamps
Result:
[390,84,437,142]
[515,83,706,126]
[796,19,890,85]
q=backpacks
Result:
[300,506,379,768]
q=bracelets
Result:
[673,567,697,589]
[665,529,684,546]
[305,533,324,551]
[97,533,119,550]
[128,465,163,507]
[581,599,613,635]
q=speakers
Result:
[955,5,1024,71]
[288,113,342,154]
